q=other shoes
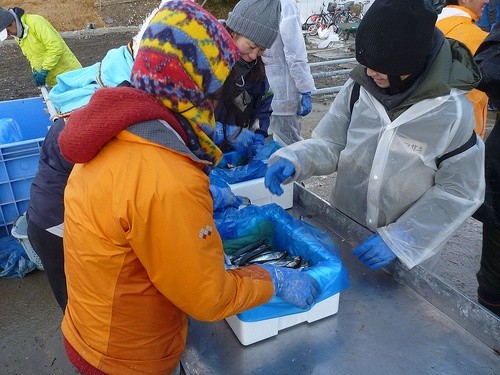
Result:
[474,292,500,316]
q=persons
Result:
[253,0,315,145]
[24,30,141,318]
[266,0,488,277]
[60,0,321,375]
[431,0,490,142]
[0,7,82,89]
[201,0,282,150]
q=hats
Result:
[355,0,438,76]
[0,8,15,31]
[127,0,238,99]
[225,0,282,50]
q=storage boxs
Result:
[211,203,350,350]
[0,96,57,243]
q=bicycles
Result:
[305,0,371,42]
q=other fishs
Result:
[230,237,311,272]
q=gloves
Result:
[351,226,416,269]
[264,159,294,196]
[31,68,49,86]
[247,132,264,158]
[296,92,312,116]
[214,121,256,154]
[263,259,320,310]
